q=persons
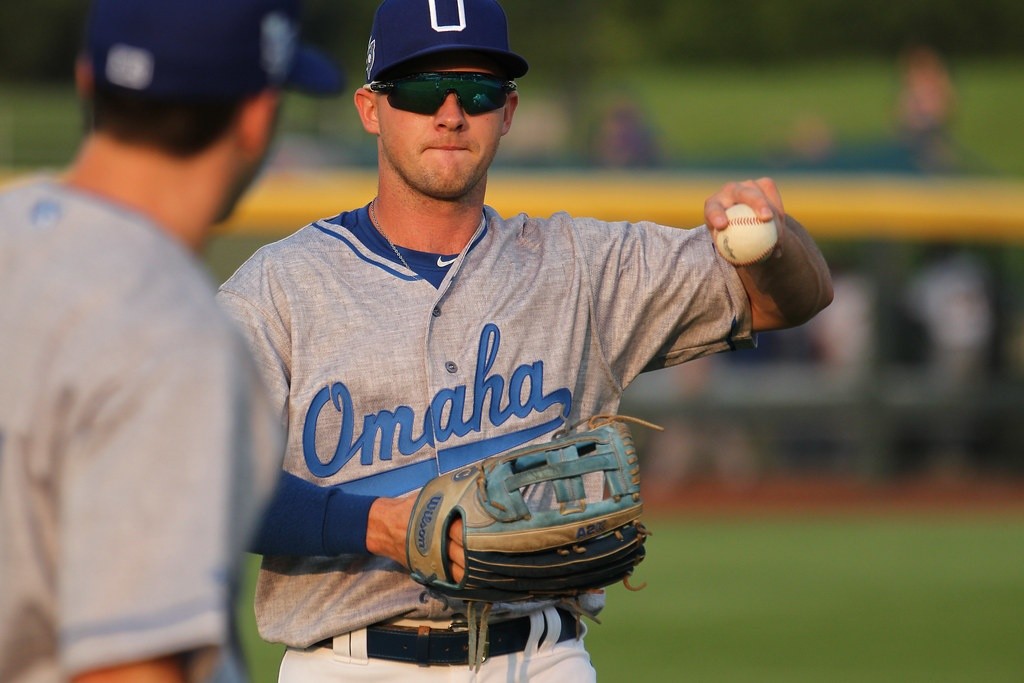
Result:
[217,0,832,683]
[585,62,1024,486]
[0,0,347,683]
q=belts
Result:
[320,604,582,666]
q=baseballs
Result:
[712,202,780,266]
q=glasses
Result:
[361,70,517,115]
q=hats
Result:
[85,0,349,99]
[367,0,530,81]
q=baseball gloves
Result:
[405,410,666,674]
